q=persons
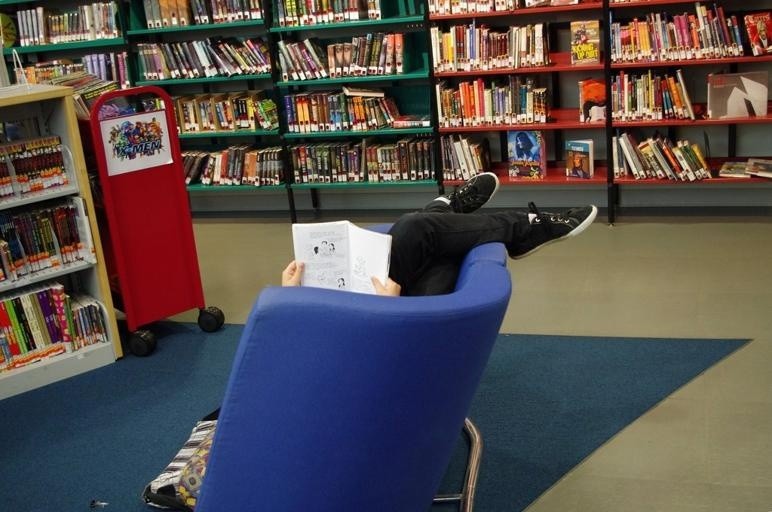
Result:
[176,172,598,509]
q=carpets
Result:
[0,320,757,510]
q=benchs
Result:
[191,224,513,510]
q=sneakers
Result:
[437,171,500,215]
[507,202,597,261]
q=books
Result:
[429,0,608,182]
[1,116,107,370]
[17,3,131,91]
[611,1,772,180]
[277,0,435,184]
[135,1,285,188]
